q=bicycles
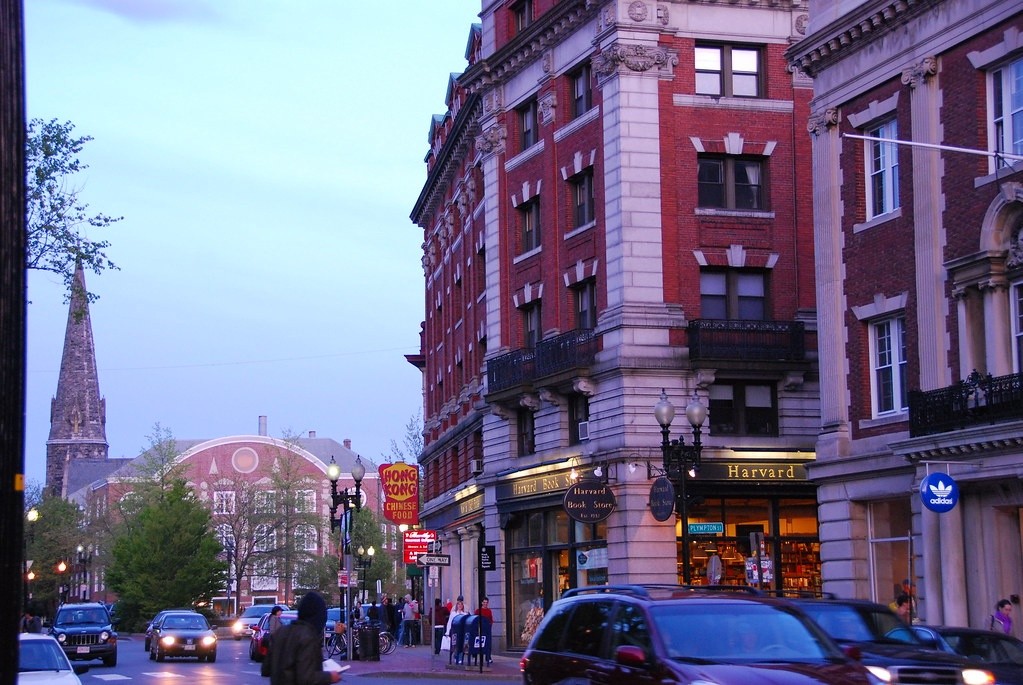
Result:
[326,618,397,658]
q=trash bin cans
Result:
[464,615,492,667]
[449,614,472,665]
[357,621,381,660]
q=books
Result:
[322,658,351,675]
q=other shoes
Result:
[458,660,462,664]
[411,645,416,648]
[489,660,493,663]
[404,645,408,648]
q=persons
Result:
[261,606,285,676]
[270,591,341,685]
[219,609,225,616]
[16,602,41,633]
[473,597,494,664]
[90,611,100,621]
[985,599,1014,636]
[241,605,246,613]
[354,594,418,648]
[428,595,466,665]
[63,613,74,623]
[892,578,917,626]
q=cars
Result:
[886,625,1023,685]
[233,603,290,641]
[17,632,89,685]
[149,612,218,663]
[255,611,298,627]
[325,607,340,646]
[248,613,297,662]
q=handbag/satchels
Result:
[441,636,450,652]
[261,632,270,648]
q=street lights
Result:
[23,508,39,632]
[654,387,706,585]
[327,453,365,660]
[77,542,94,603]
[358,544,375,604]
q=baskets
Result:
[334,624,345,634]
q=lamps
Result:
[627,452,662,474]
[592,455,612,479]
[568,456,586,480]
[689,463,697,479]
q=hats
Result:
[457,595,464,601]
[380,595,387,602]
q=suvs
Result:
[519,584,895,685]
[52,600,118,667]
[145,608,197,652]
[767,590,997,685]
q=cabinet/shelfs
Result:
[677,535,824,599]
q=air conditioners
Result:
[578,421,589,441]
[470,458,486,474]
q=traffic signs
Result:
[417,553,452,567]
